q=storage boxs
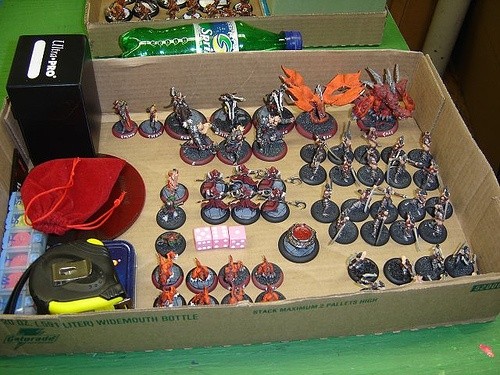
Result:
[0,48,500,357]
[84,0,388,58]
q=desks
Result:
[0,0,500,374]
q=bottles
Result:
[118,21,303,59]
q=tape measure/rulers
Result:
[28,237,134,316]
